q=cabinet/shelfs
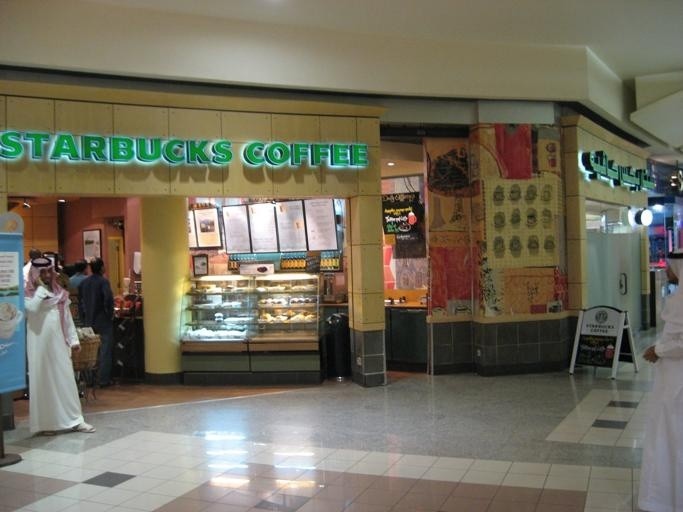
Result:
[181,273,323,386]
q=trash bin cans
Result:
[325,313,351,383]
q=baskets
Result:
[71,335,103,369]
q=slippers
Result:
[72,423,96,433]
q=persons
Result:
[24,257,95,435]
[78,256,118,387]
[23,248,90,328]
[638,247,683,512]
